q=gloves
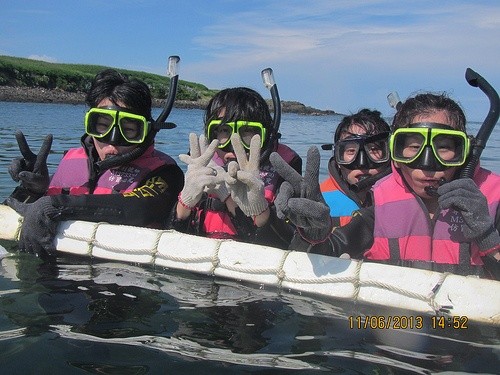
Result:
[268,145,333,245]
[225,132,269,217]
[198,153,232,203]
[4,196,60,252]
[176,133,224,210]
[435,176,500,257]
[7,129,58,194]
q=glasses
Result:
[206,121,265,153]
[83,107,151,146]
[332,133,390,166]
[389,127,468,169]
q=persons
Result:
[164,86,303,250]
[0,69,184,261]
[319,109,392,231]
[270,94,500,283]
